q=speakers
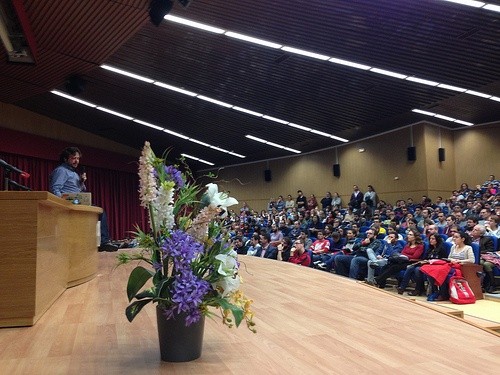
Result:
[333,165,340,176]
[407,147,416,161]
[439,148,445,161]
[265,170,271,181]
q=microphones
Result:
[8,179,32,191]
[81,170,83,182]
[0,158,30,178]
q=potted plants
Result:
[156,305,205,363]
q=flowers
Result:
[109,141,258,334]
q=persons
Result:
[110,174,499,301]
[51,146,118,253]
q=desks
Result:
[0,191,104,328]
